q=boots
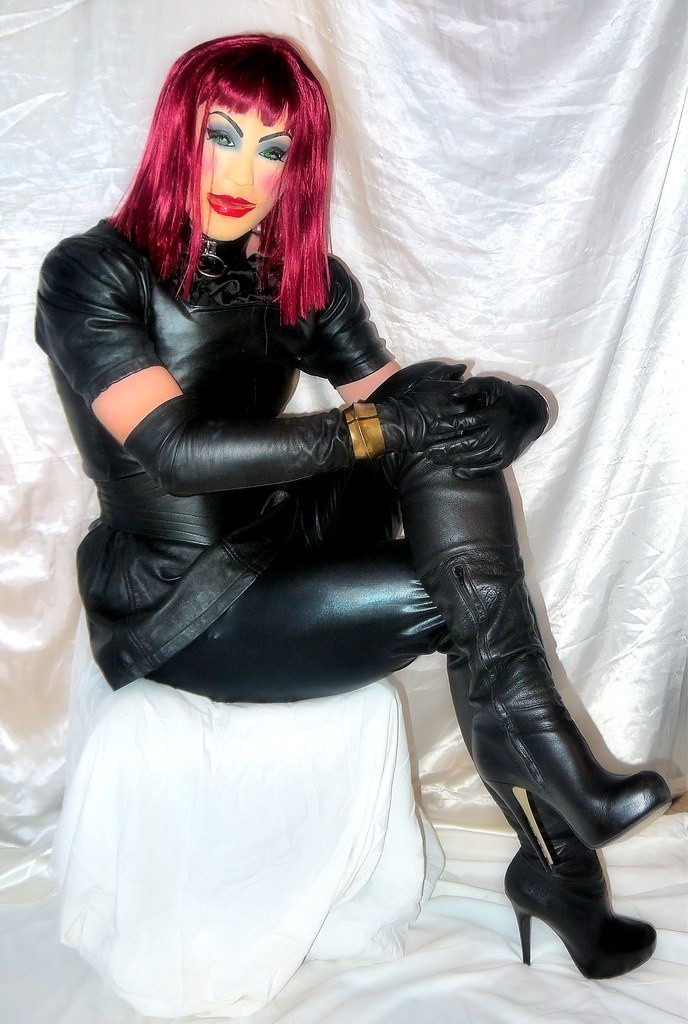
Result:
[420,544,671,877]
[480,774,657,979]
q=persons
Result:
[35,32,671,980]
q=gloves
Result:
[358,362,547,483]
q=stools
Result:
[54,680,447,1022]
